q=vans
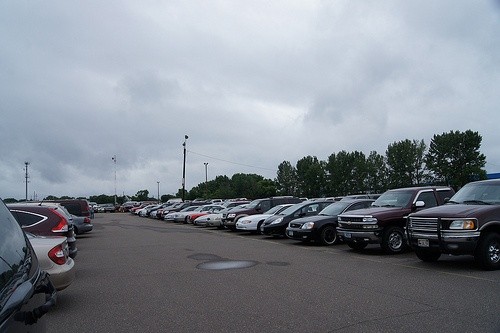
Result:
[37,199,94,220]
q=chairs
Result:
[309,207,312,211]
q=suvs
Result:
[0,197,59,332]
[4,202,77,257]
[220,196,303,233]
[405,178,500,271]
[334,186,456,256]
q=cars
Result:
[89,201,116,213]
[286,199,376,247]
[300,194,398,207]
[235,204,298,236]
[70,214,93,235]
[23,229,76,292]
[259,199,340,239]
[205,207,235,230]
[119,197,252,228]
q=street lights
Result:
[181,134,188,202]
[203,162,208,184]
[157,181,160,199]
